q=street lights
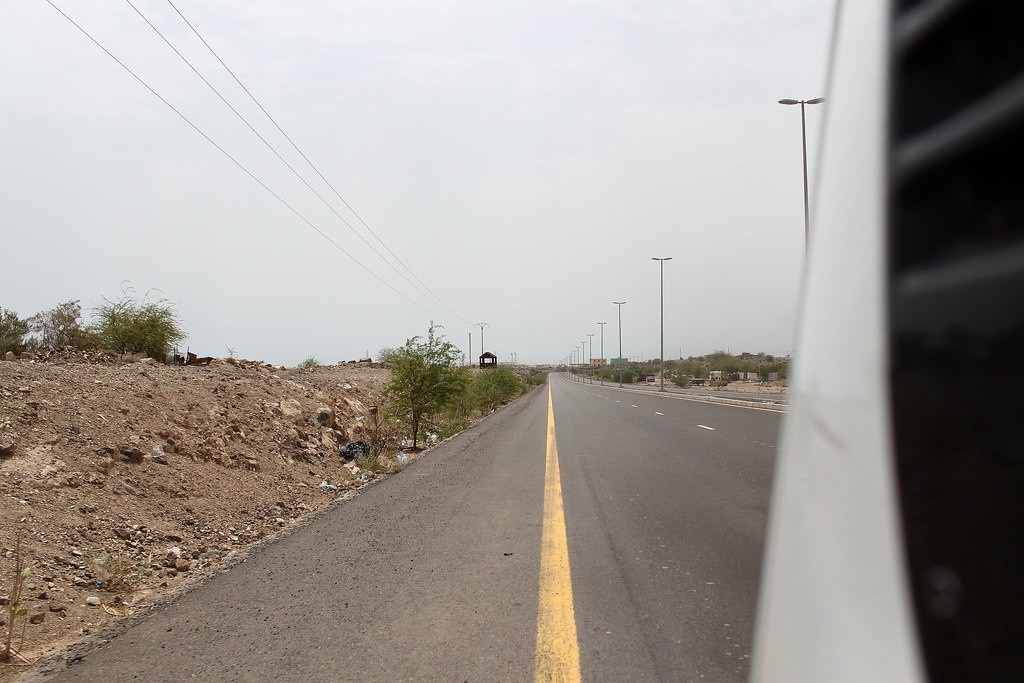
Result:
[474,322,490,362]
[597,322,606,385]
[776,94,825,264]
[651,255,671,392]
[611,300,626,387]
[560,332,593,384]
